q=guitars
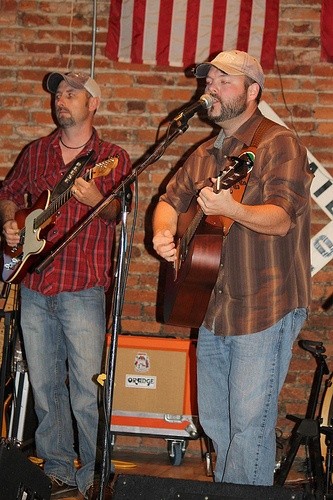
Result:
[162,154,250,329]
[1,153,120,285]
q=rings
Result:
[74,189,78,193]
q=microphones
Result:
[174,94,213,123]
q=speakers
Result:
[102,333,203,437]
[0,446,53,500]
[111,473,315,500]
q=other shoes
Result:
[50,477,112,500]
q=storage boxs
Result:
[106,333,199,439]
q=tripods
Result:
[272,340,330,494]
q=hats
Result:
[194,49,264,93]
[46,72,101,111]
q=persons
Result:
[152,49,310,486]
[0,72,132,500]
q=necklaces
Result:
[58,135,88,149]
